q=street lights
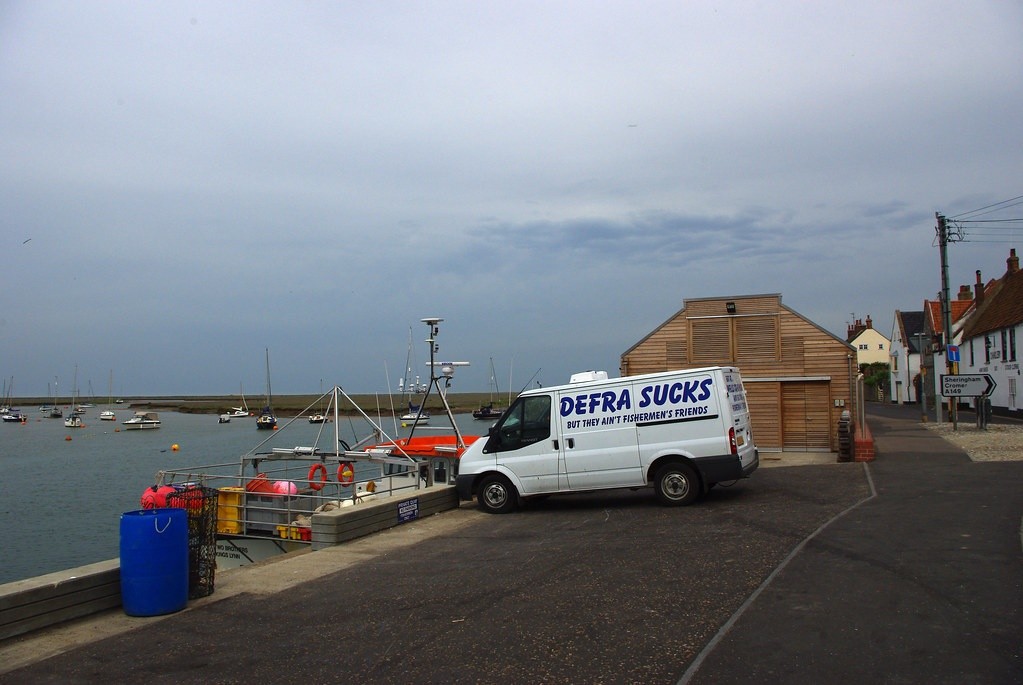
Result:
[914,333,927,422]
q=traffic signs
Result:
[941,374,997,398]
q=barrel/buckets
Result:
[246,473,273,493]
[119,508,189,617]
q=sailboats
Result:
[78,379,97,408]
[256,347,277,429]
[38,382,52,410]
[0,375,27,422]
[100,369,115,420]
[65,361,83,427]
[308,378,329,423]
[50,375,62,418]
[399,325,430,425]
[226,383,249,418]
[74,387,86,414]
[471,358,504,419]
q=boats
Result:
[122,412,161,429]
[149,318,479,571]
[218,413,231,423]
[115,399,124,404]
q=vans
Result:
[455,368,759,512]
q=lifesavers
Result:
[338,463,354,487]
[309,464,327,490]
